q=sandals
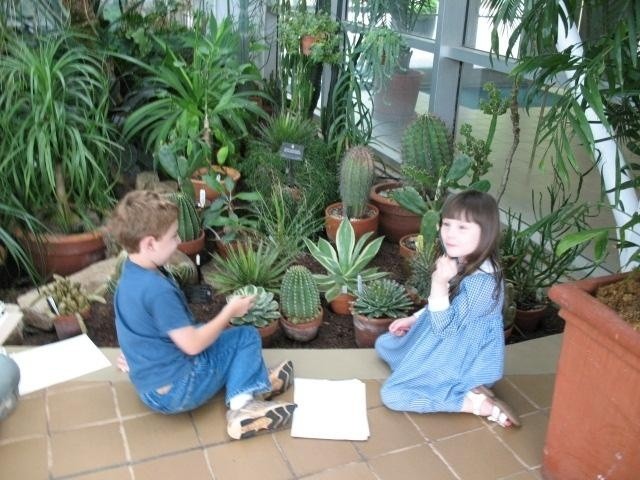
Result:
[465,385,521,428]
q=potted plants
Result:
[0,0,614,353]
[491,1,640,480]
[492,2,640,478]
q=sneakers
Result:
[225,397,297,440]
[253,360,295,401]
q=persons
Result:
[110,190,297,440]
[374,190,523,427]
